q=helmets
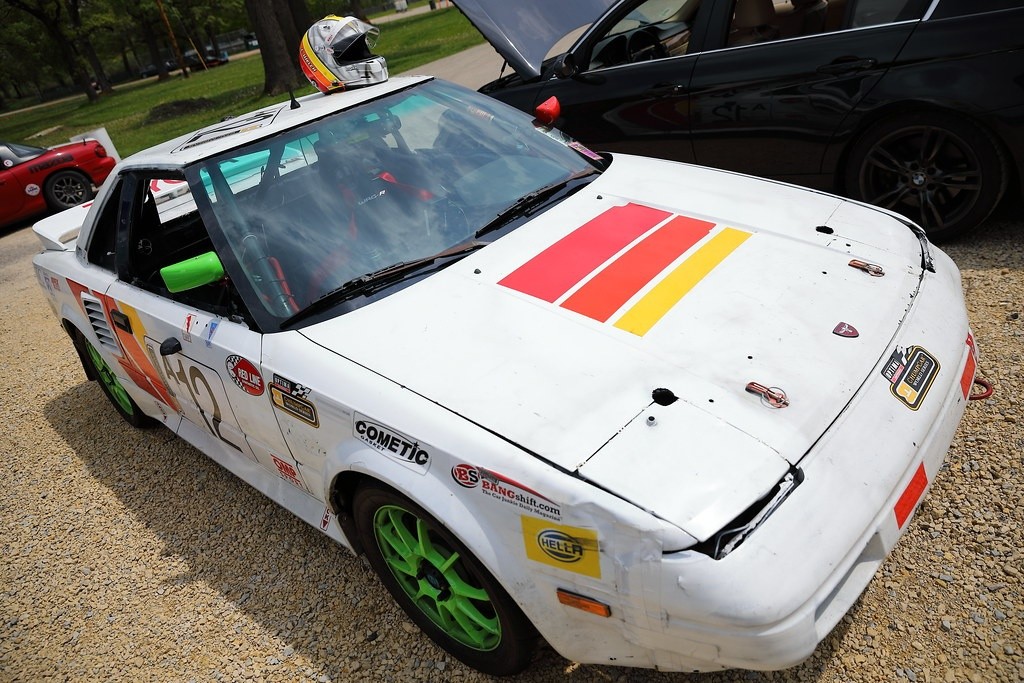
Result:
[299,13,388,96]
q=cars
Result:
[31,71,982,683]
[189,49,229,70]
[431,0,1024,247]
[138,59,176,80]
[0,139,119,235]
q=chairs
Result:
[315,126,452,263]
[783,0,828,40]
[253,187,371,319]
[726,0,781,48]
